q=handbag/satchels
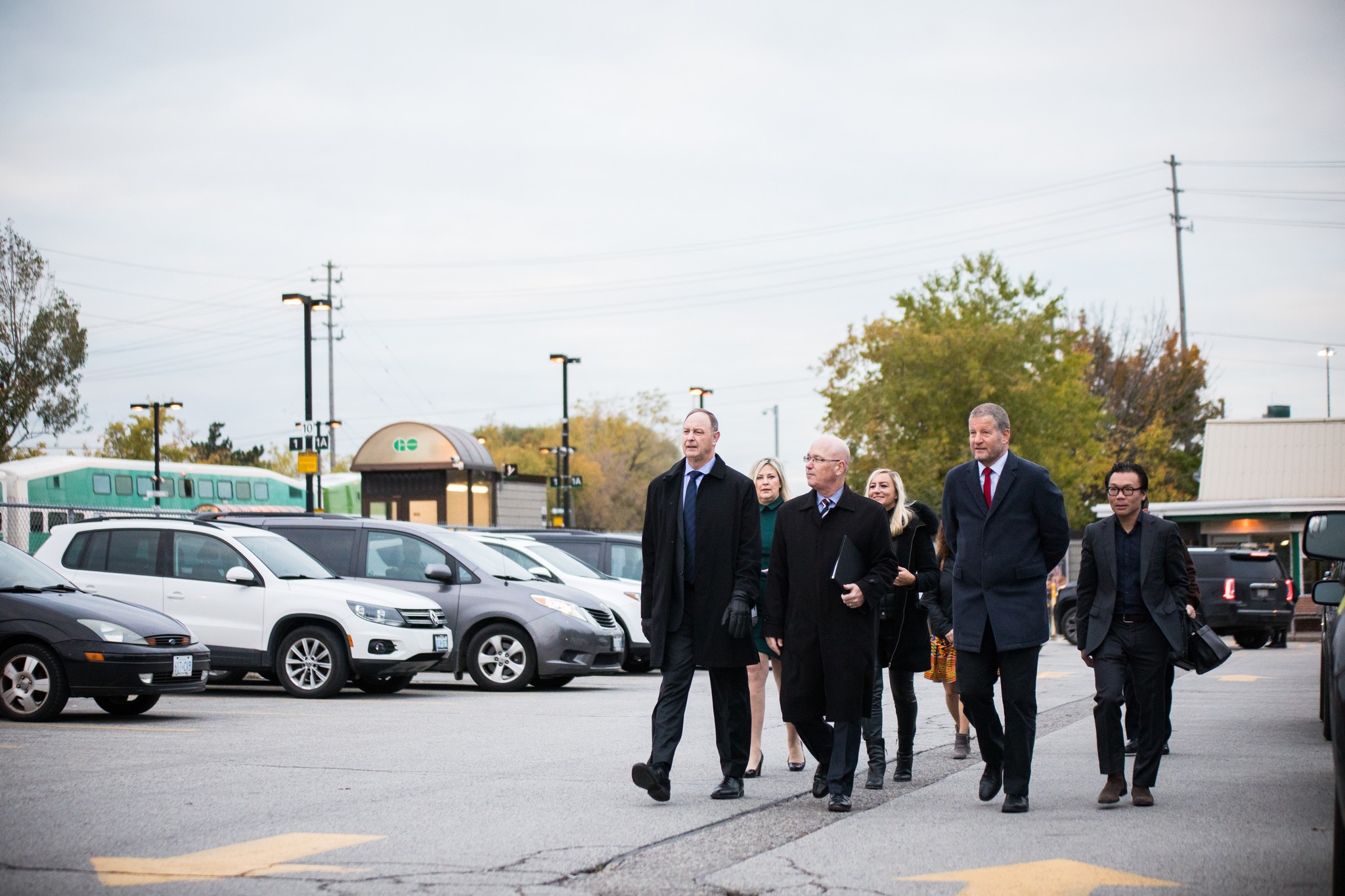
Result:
[1188,617,1232,675]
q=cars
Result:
[1303,509,1345,896]
[0,537,211,722]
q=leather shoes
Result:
[979,762,1003,801]
[828,793,852,811]
[1162,742,1169,755]
[1002,794,1029,813]
[1125,738,1138,755]
[711,775,744,799]
[1098,771,1127,803]
[632,763,671,801]
[1131,784,1154,806]
[812,762,830,798]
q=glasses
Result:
[803,456,840,464]
[1106,487,1142,496]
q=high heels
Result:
[788,740,806,771]
[743,750,764,778]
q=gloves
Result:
[722,590,753,638]
[641,618,652,643]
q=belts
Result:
[1112,613,1150,623]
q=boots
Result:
[953,724,971,759]
[866,738,886,789]
[894,734,915,782]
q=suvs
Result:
[368,532,656,673]
[192,509,629,694]
[1049,547,1298,649]
[29,513,453,701]
[489,530,648,583]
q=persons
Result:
[942,403,1069,813]
[743,459,806,776]
[1077,464,1201,805]
[766,434,895,811]
[862,468,999,790]
[631,409,761,802]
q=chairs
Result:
[177,540,224,579]
[385,554,401,579]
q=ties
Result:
[684,471,703,584]
[983,468,993,509]
[820,498,832,519]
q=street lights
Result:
[294,420,343,514]
[548,354,583,530]
[129,403,185,517]
[763,404,779,460]
[280,293,333,514]
[689,386,712,410]
[1316,345,1337,420]
[539,445,566,530]
